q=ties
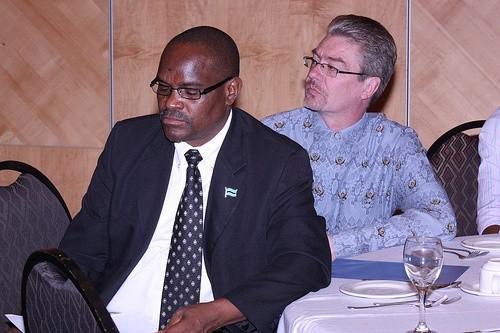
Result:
[159,149,204,331]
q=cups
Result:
[479,258,500,293]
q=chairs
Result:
[428,119,486,237]
[22,247,120,333]
[0,159,72,333]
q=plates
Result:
[461,280,500,296]
[461,237,500,250]
[338,278,419,298]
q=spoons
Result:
[362,278,461,290]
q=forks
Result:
[373,296,463,305]
[444,249,480,259]
[348,296,449,310]
[442,245,490,257]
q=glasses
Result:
[302,56,369,78]
[150,77,236,100]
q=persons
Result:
[261,14,457,262]
[57,25,331,333]
[476,107,500,234]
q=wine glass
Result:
[402,235,444,333]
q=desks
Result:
[276,234,500,333]
